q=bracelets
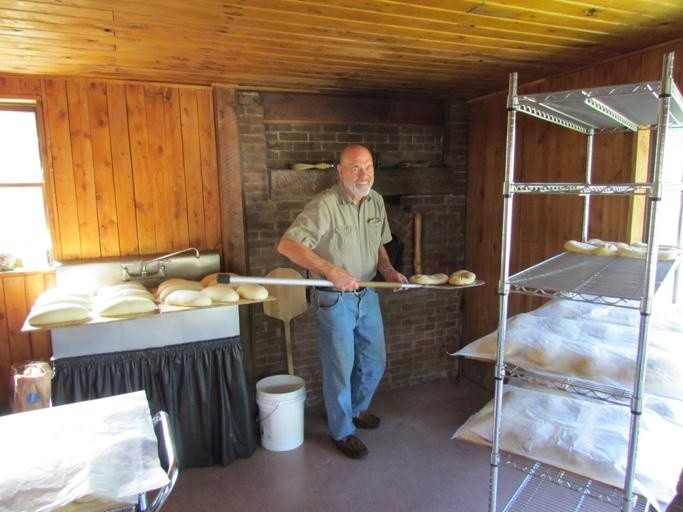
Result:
[382,265,395,279]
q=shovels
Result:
[217,274,486,290]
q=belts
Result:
[315,285,367,293]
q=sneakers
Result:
[328,434,370,460]
[353,410,380,431]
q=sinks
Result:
[51,250,240,358]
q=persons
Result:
[276,142,408,459]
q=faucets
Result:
[140,247,200,279]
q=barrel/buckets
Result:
[255,374,307,452]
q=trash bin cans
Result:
[10,360,51,413]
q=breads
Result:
[27,272,270,325]
[409,269,477,286]
[482,238,678,386]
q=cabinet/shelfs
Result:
[485,47,682,509]
